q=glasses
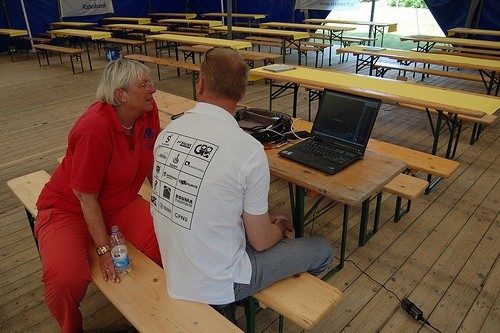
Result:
[128,80,152,88]
[203,45,235,69]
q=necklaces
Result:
[121,124,133,130]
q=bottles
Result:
[109,226,131,275]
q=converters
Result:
[400,298,423,322]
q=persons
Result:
[151,47,334,309]
[33,59,163,333]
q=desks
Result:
[0,13,500,268]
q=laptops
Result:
[278,88,382,175]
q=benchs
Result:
[7,27,499,333]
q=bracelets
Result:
[97,245,110,255]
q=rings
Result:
[107,270,110,272]
